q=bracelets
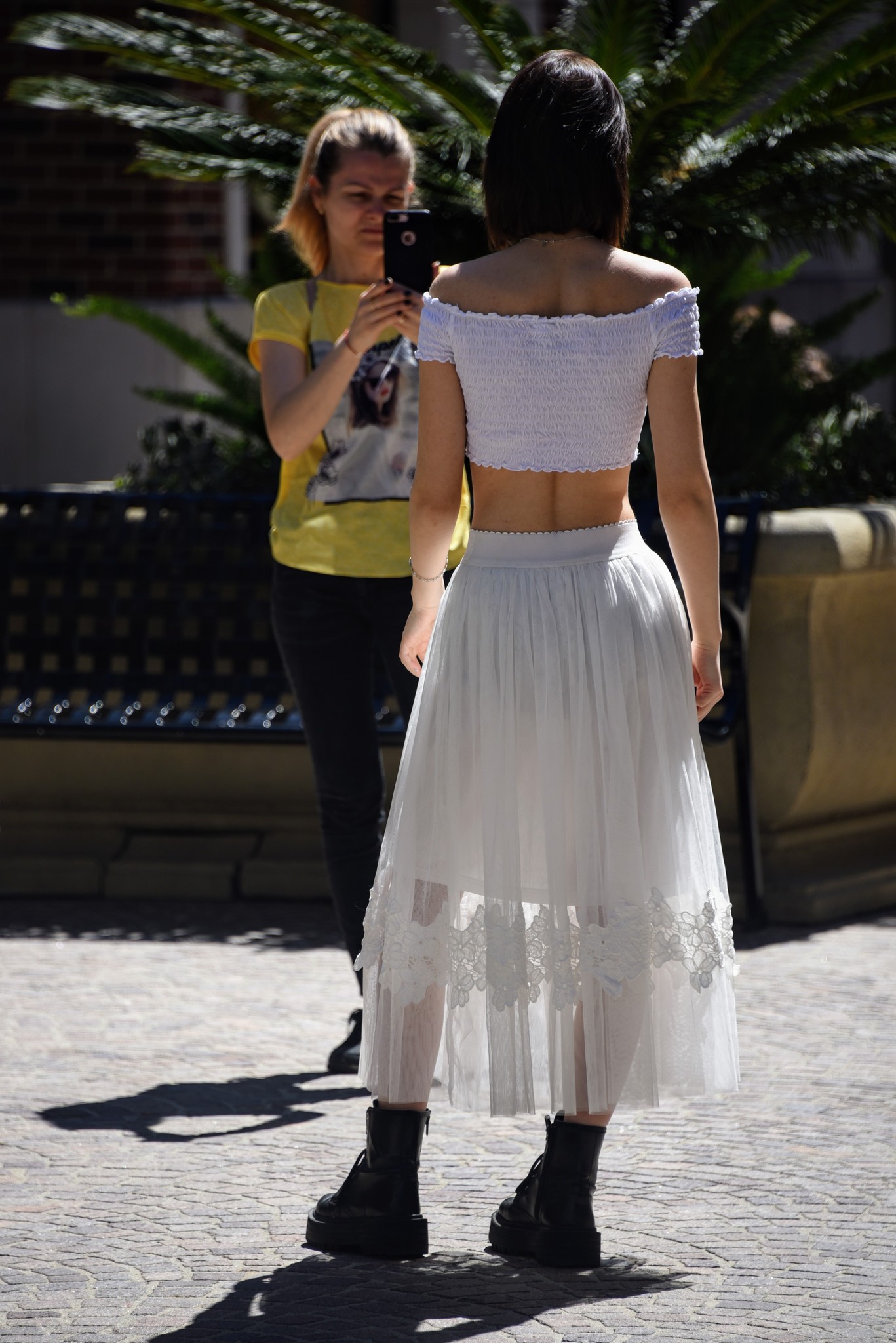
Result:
[344,331,363,358]
[409,557,448,583]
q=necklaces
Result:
[520,235,603,246]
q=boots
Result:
[307,1098,431,1258]
[487,1109,607,1268]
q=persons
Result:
[247,109,471,1075]
[730,306,878,477]
[308,47,737,1267]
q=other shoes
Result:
[327,1003,365,1071]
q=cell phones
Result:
[383,208,435,315]
